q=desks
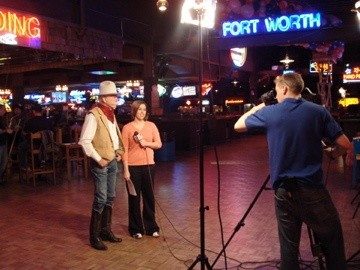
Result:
[60,142,88,180]
[157,119,201,153]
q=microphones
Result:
[134,131,146,149]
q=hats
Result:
[92,81,122,96]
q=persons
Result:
[78,81,125,250]
[122,101,162,239]
[234,73,352,270]
[8,104,51,176]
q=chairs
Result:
[18,122,90,187]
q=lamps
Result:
[156,0,169,12]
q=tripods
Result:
[187,14,213,269]
[210,173,326,270]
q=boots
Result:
[90,208,108,250]
[101,204,122,242]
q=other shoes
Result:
[133,233,142,238]
[40,159,47,168]
[153,231,159,237]
[0,180,8,186]
